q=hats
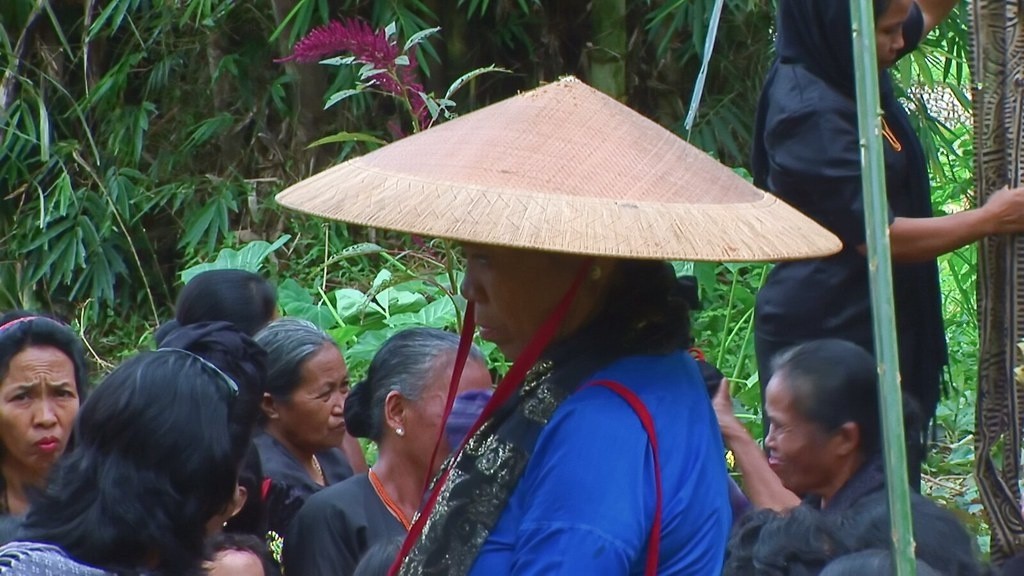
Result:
[275,73,843,264]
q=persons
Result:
[690,338,1024,576]
[752,1,1023,498]
[0,267,493,576]
[406,92,734,576]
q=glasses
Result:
[153,345,239,399]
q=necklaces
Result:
[370,471,412,531]
[311,454,324,480]
